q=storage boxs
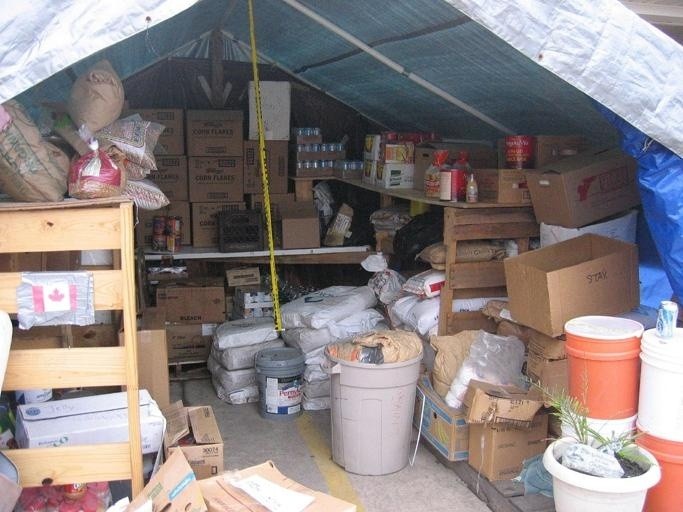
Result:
[411,84,643,480]
[1,80,360,511]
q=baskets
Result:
[216,211,265,253]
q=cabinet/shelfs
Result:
[291,176,540,356]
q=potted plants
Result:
[520,367,662,512]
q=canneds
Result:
[151,215,182,252]
[439,168,466,203]
[297,159,363,170]
[296,143,344,153]
[364,129,440,152]
[655,301,679,338]
[291,127,321,137]
[504,135,534,170]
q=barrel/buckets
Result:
[563,316,644,420]
[637,327,683,443]
[253,327,308,422]
[560,414,638,442]
[634,428,682,512]
[318,349,424,476]
